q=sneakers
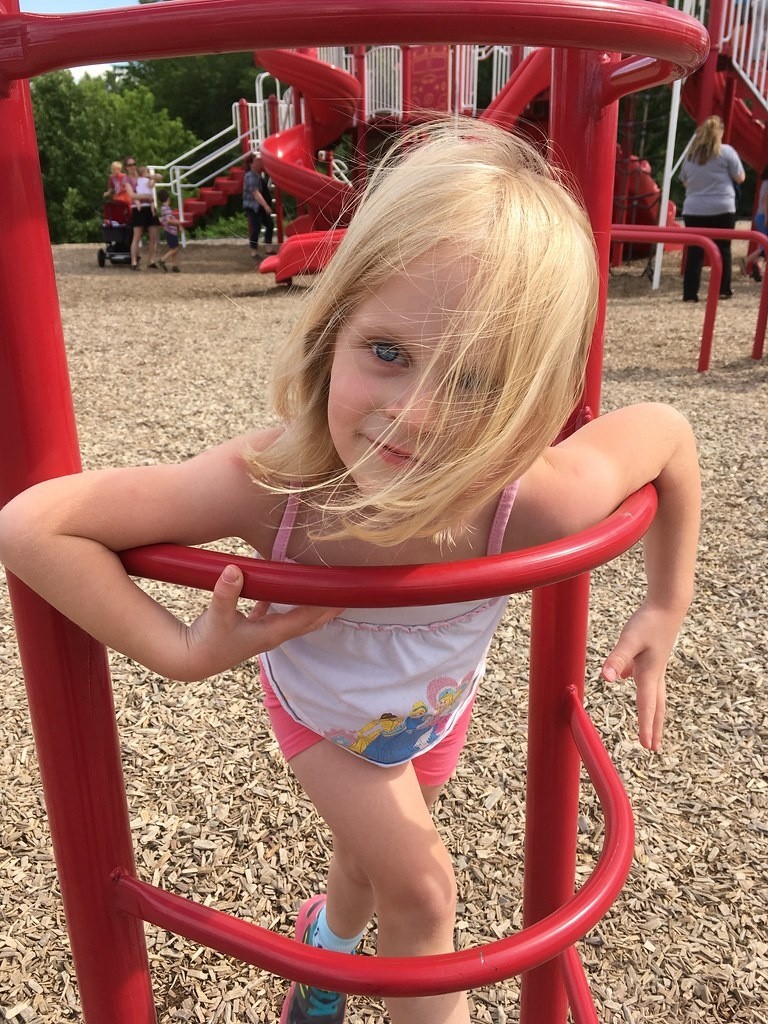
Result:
[276,890,348,1024]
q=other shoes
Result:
[264,250,276,257]
[147,263,157,268]
[751,261,764,282]
[130,265,140,270]
[738,257,748,278]
[250,253,264,262]
[719,288,734,299]
[156,259,168,273]
[683,294,698,302]
[171,264,181,273]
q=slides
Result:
[257,45,359,285]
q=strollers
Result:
[96,195,142,267]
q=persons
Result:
[680,115,745,303]
[243,155,277,263]
[157,190,184,273]
[103,157,160,270]
[1,118,701,1024]
[740,163,768,282]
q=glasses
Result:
[126,163,136,167]
